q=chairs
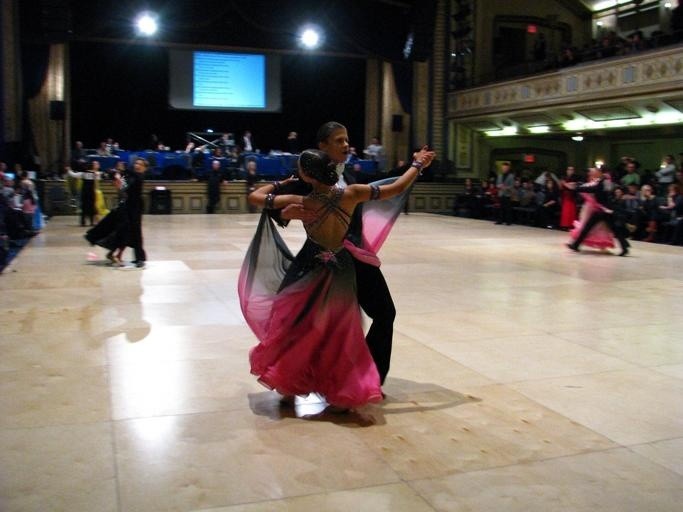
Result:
[83,152,380,179]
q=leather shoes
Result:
[566,242,579,252]
[135,261,144,267]
[618,250,628,257]
[130,260,141,264]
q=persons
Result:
[267,121,437,400]
[64,132,410,267]
[530,0,683,77]
[247,149,436,417]
[450,155,683,257]
[0,161,49,266]
